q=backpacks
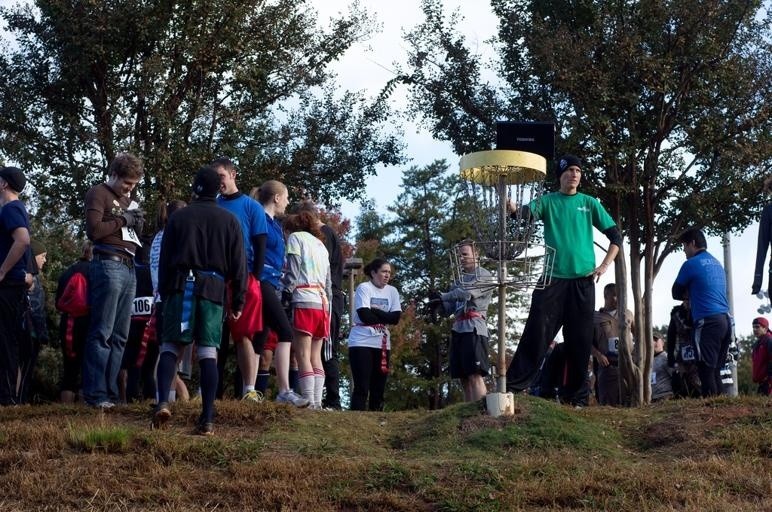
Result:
[55,262,89,318]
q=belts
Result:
[92,253,135,269]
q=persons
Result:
[24,236,49,403]
[420,238,494,402]
[531,282,742,402]
[499,154,623,407]
[673,230,732,401]
[750,317,771,396]
[351,257,402,411]
[0,167,35,407]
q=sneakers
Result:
[241,387,260,404]
[199,413,215,435]
[276,390,311,410]
[155,401,172,420]
[84,399,117,412]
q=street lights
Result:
[342,253,364,333]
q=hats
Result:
[191,167,221,199]
[556,154,582,180]
[752,317,772,334]
[30,237,48,256]
[652,330,666,341]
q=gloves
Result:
[120,209,149,239]
